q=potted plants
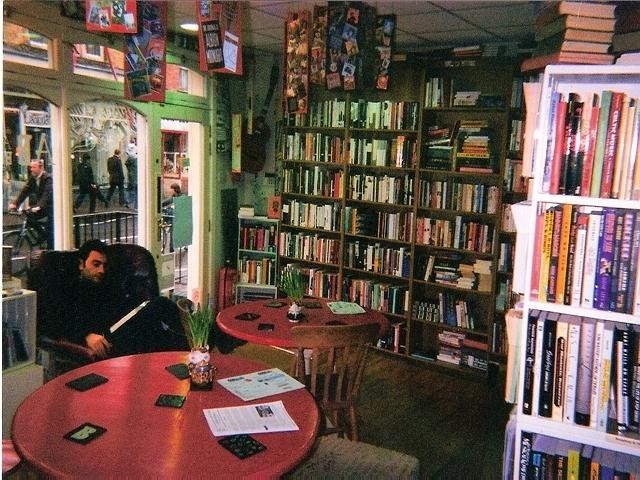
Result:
[186,293,214,365]
[280,265,307,311]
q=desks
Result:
[12,351,322,480]
[216,298,389,376]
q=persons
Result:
[12,146,22,180]
[9,158,54,250]
[125,156,137,211]
[39,238,191,362]
[105,149,127,209]
[73,152,98,213]
[161,182,185,253]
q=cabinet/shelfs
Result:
[513,64,640,480]
[237,217,278,287]
[279,56,524,386]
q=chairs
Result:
[290,322,380,442]
[30,243,180,379]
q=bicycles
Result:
[2,207,46,276]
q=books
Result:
[523,310,640,439]
[496,73,545,479]
[531,201,639,314]
[203,400,300,437]
[2,273,32,370]
[520,1,640,76]
[238,225,277,303]
[239,196,280,218]
[521,431,640,480]
[544,91,640,200]
[411,43,506,370]
[278,98,345,300]
[342,99,420,354]
[216,367,305,403]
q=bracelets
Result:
[15,153,19,157]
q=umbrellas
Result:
[95,188,111,207]
